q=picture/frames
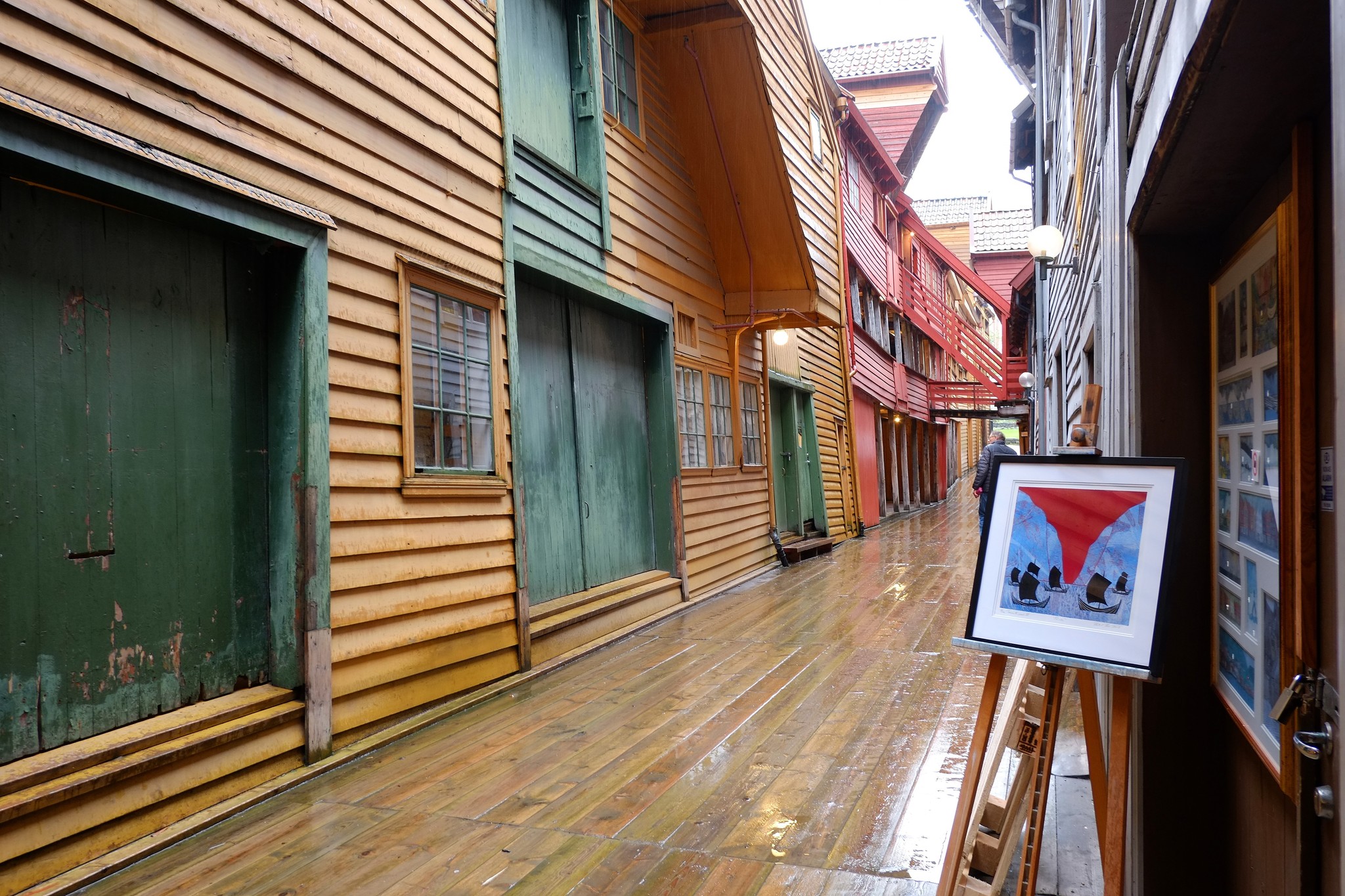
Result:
[964,454,1191,675]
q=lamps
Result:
[1019,372,1037,392]
[1027,225,1078,280]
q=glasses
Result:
[988,436,996,440]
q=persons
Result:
[972,431,1018,539]
